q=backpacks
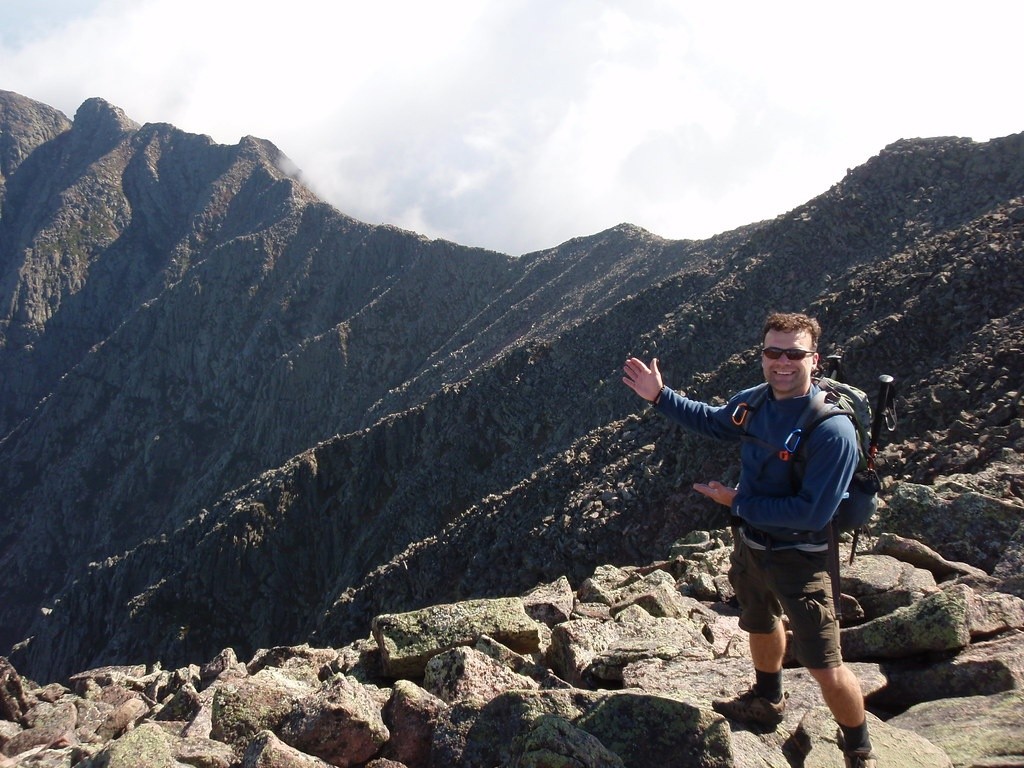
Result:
[735,375,883,530]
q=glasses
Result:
[762,346,815,361]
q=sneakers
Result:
[712,683,785,726]
[842,737,878,768]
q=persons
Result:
[620,314,886,768]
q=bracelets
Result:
[652,387,665,404]
